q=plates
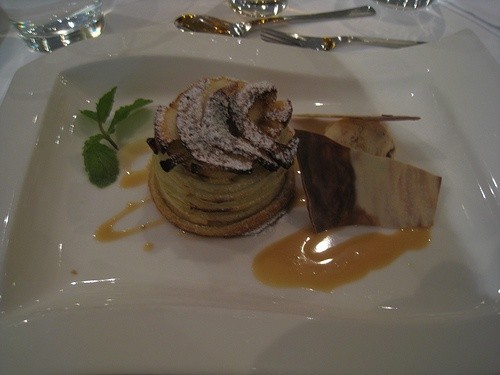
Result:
[1,29,499,373]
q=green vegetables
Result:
[78,85,152,187]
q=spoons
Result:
[174,6,376,38]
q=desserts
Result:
[147,76,301,236]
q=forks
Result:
[259,26,427,51]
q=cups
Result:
[1,0,105,51]
[230,0,288,20]
